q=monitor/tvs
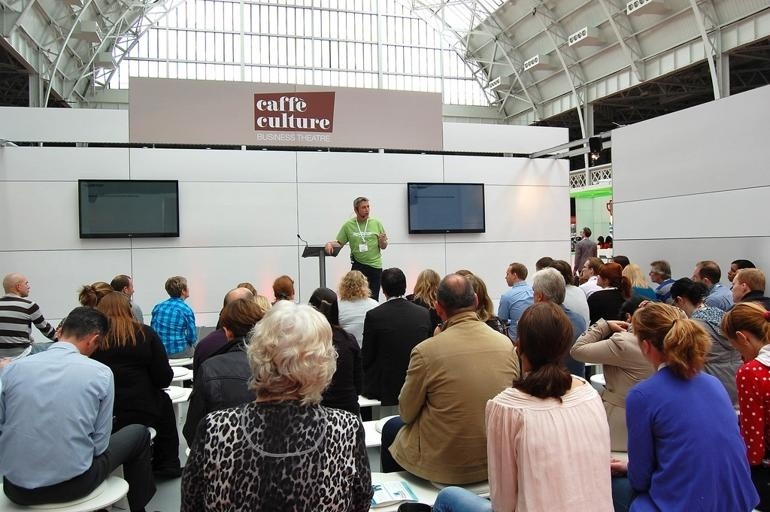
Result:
[407,181,485,234]
[78,179,180,239]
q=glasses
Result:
[582,265,587,269]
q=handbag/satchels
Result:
[573,272,580,287]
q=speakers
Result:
[589,136,603,154]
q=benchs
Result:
[369,472,490,512]
[111,427,157,510]
[362,420,392,448]
[169,367,193,388]
[358,394,382,408]
[169,357,193,367]
[164,386,192,425]
[1,475,130,512]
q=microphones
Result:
[356,207,365,218]
[297,233,308,247]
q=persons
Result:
[572,227,613,276]
[361,257,770,512]
[180,271,380,512]
[1,273,199,512]
[324,196,388,302]
